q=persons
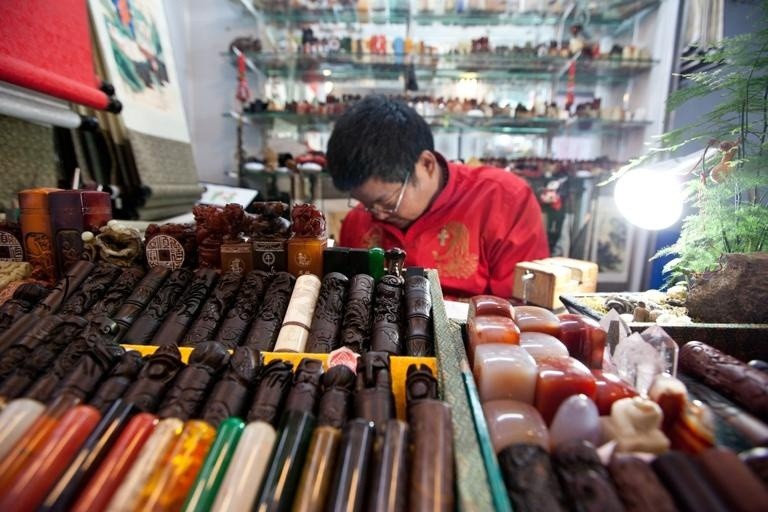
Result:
[326,94,551,301]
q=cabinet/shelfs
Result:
[222,0,677,178]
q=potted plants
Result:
[559,0,768,362]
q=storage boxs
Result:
[384,266,494,511]
[449,317,768,512]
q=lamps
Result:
[612,146,721,230]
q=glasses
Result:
[347,170,412,216]
